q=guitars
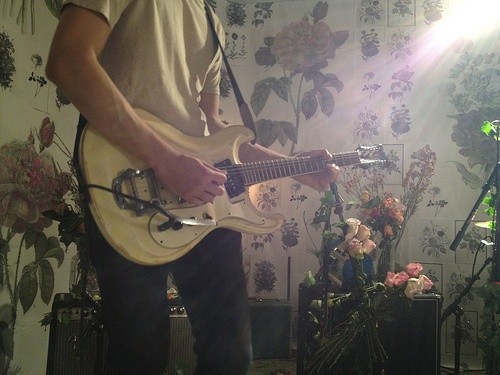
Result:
[75,104,389,267]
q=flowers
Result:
[300,186,432,374]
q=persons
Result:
[45,0,340,375]
